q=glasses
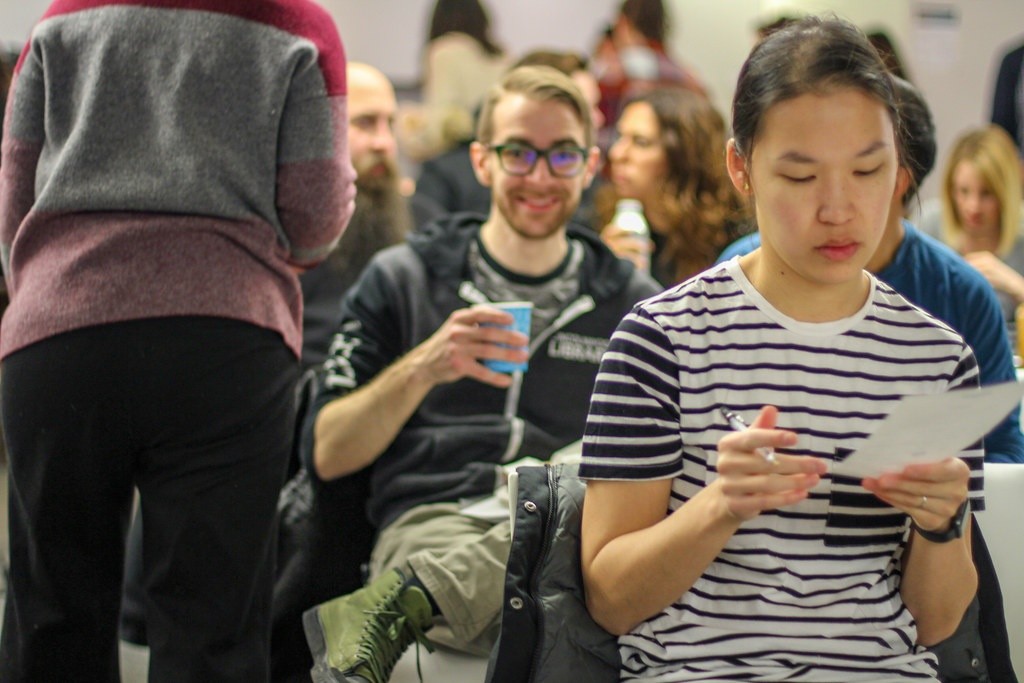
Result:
[488,143,587,179]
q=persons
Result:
[0,0,359,681]
[574,11,989,682]
[301,66,666,683]
[120,0,1024,645]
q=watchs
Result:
[910,496,972,543]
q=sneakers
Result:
[303,570,436,683]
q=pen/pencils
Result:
[720,405,781,466]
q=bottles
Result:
[610,198,651,276]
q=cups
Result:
[471,301,534,373]
